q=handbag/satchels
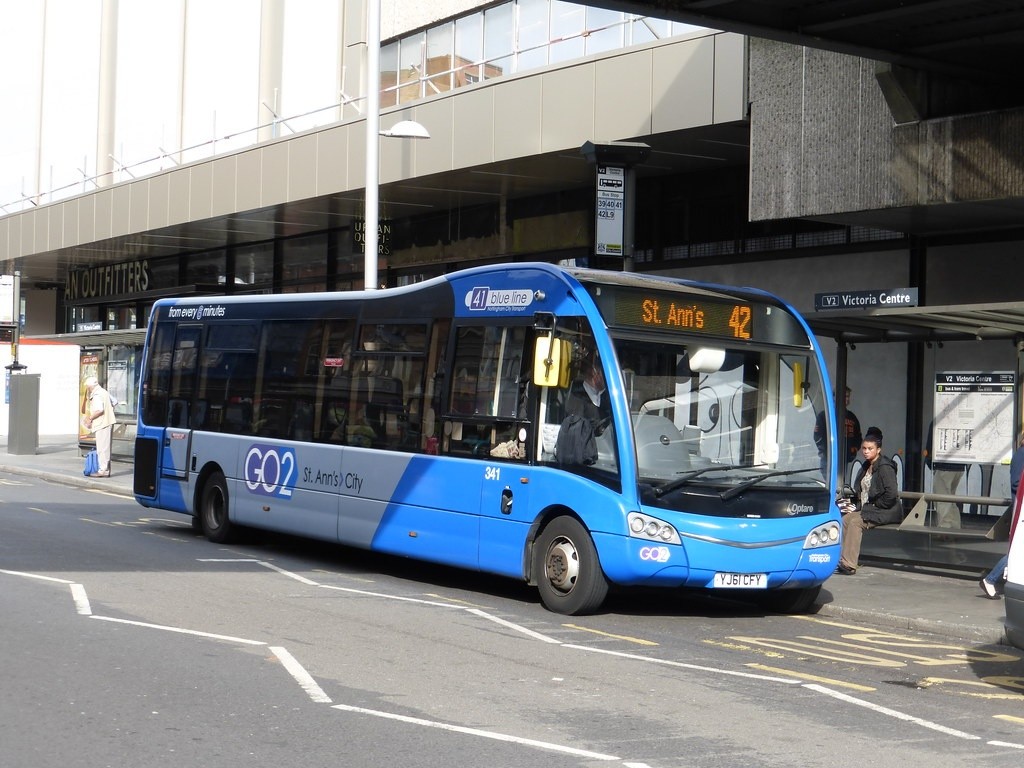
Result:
[836,484,860,515]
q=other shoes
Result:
[979,579,1001,599]
[833,562,856,575]
[90,471,110,477]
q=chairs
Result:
[176,365,623,466]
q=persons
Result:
[837,427,903,573]
[983,431,1024,598]
[814,385,862,481]
[84,376,117,478]
[564,356,612,433]
[924,419,965,543]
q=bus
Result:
[132,261,848,617]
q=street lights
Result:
[363,119,433,293]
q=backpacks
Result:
[84,448,98,476]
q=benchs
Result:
[876,493,1016,542]
